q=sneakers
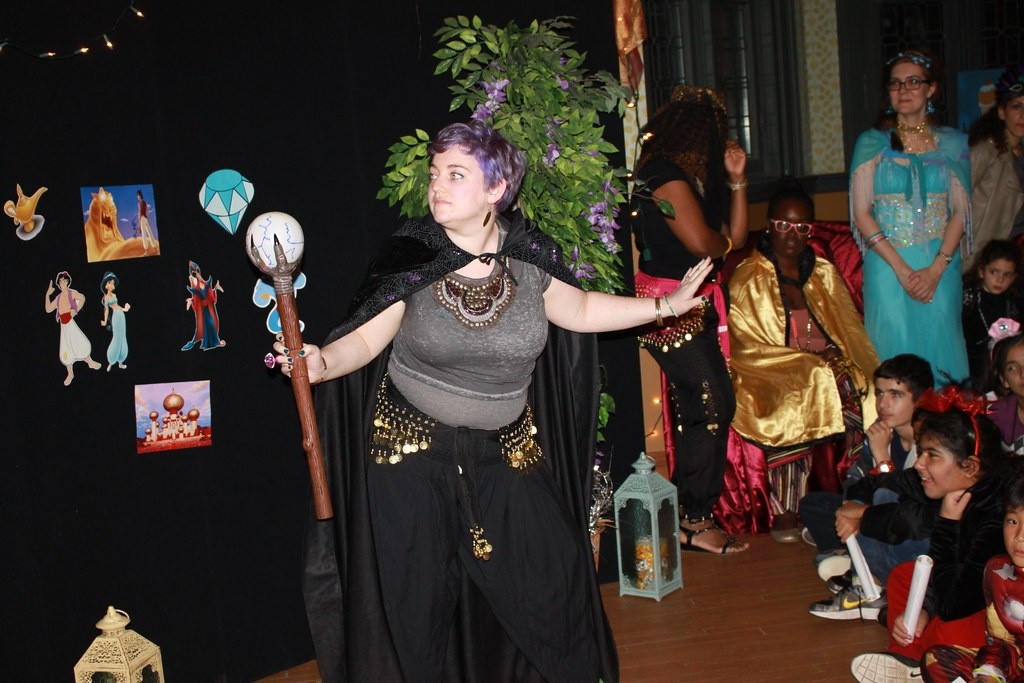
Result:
[825,569,853,594]
[809,585,887,622]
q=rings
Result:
[685,275,689,280]
[264,352,279,368]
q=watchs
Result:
[869,460,896,475]
[937,252,951,261]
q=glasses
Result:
[885,78,930,92]
[768,217,817,234]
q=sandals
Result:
[680,519,748,555]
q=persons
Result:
[960,71,1024,281]
[265,122,715,683]
[727,192,1024,683]
[849,50,970,391]
[628,82,750,554]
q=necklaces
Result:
[788,311,812,353]
[898,121,929,155]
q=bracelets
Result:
[724,236,733,255]
[655,297,664,326]
[664,291,679,317]
[726,182,748,191]
[864,231,888,249]
[311,357,327,385]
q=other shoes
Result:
[800,517,817,543]
[770,527,799,543]
[818,554,853,581]
[878,604,888,627]
[851,651,925,683]
